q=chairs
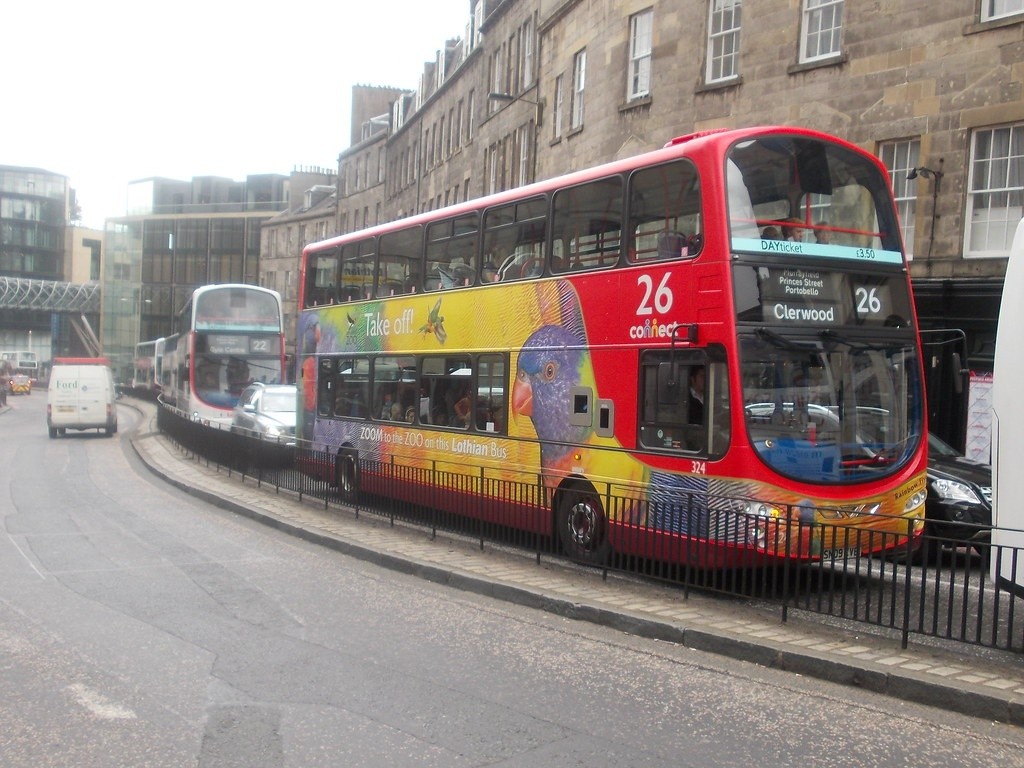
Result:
[658,229,701,260]
[309,253,585,307]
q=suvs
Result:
[230,380,296,446]
[739,401,993,568]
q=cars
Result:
[9,374,33,395]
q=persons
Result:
[781,217,805,243]
[352,357,503,431]
[431,252,465,287]
[813,222,833,244]
[0,353,14,406]
[763,227,780,241]
[774,375,823,428]
[481,239,522,282]
[673,363,730,451]
[318,359,344,414]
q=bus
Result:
[133,336,178,402]
[179,284,285,429]
[0,351,38,383]
[294,126,970,573]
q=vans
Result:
[987,222,1024,596]
[47,357,118,436]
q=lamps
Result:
[906,167,943,181]
[487,93,543,127]
